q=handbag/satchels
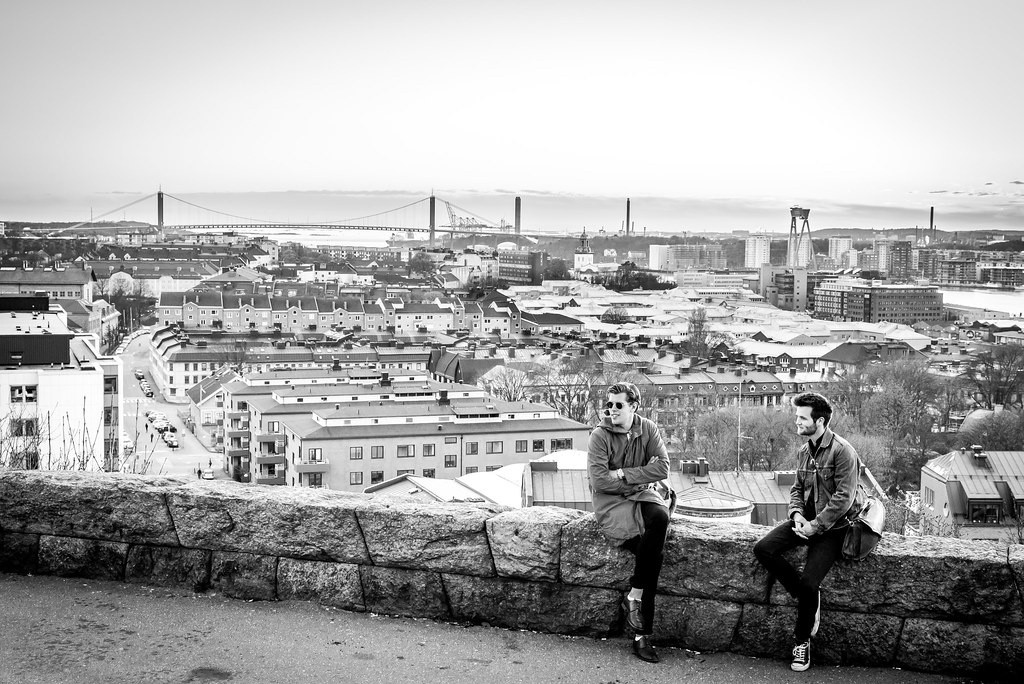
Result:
[842,496,885,560]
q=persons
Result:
[752,392,866,672]
[584,381,679,664]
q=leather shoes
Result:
[621,593,644,630]
[632,636,659,662]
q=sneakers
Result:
[809,586,821,636]
[791,638,810,672]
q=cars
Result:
[202,470,215,480]
[146,411,179,447]
[123,429,135,454]
[135,370,154,397]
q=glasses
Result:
[606,402,632,409]
